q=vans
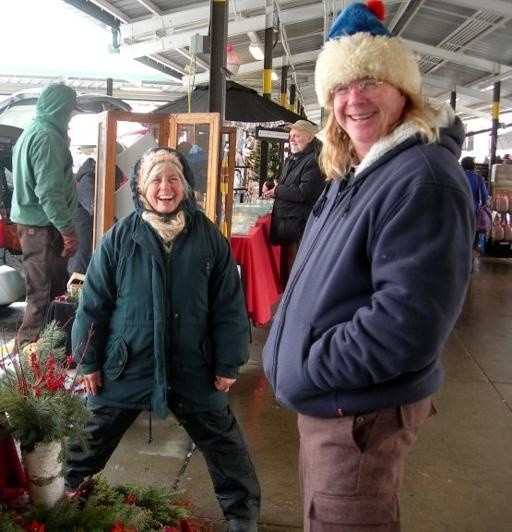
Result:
[0,87,132,306]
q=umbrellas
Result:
[139,80,320,128]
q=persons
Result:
[51,141,125,300]
[60,147,260,532]
[262,0,476,531]
[461,156,491,274]
[261,119,327,292]
[9,82,79,358]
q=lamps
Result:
[248,43,265,60]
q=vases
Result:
[21,440,66,507]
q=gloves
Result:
[61,229,79,257]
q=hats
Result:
[315,0,421,109]
[284,119,314,135]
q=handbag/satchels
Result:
[477,206,492,232]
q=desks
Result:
[231,197,283,344]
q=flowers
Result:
[0,310,99,478]
